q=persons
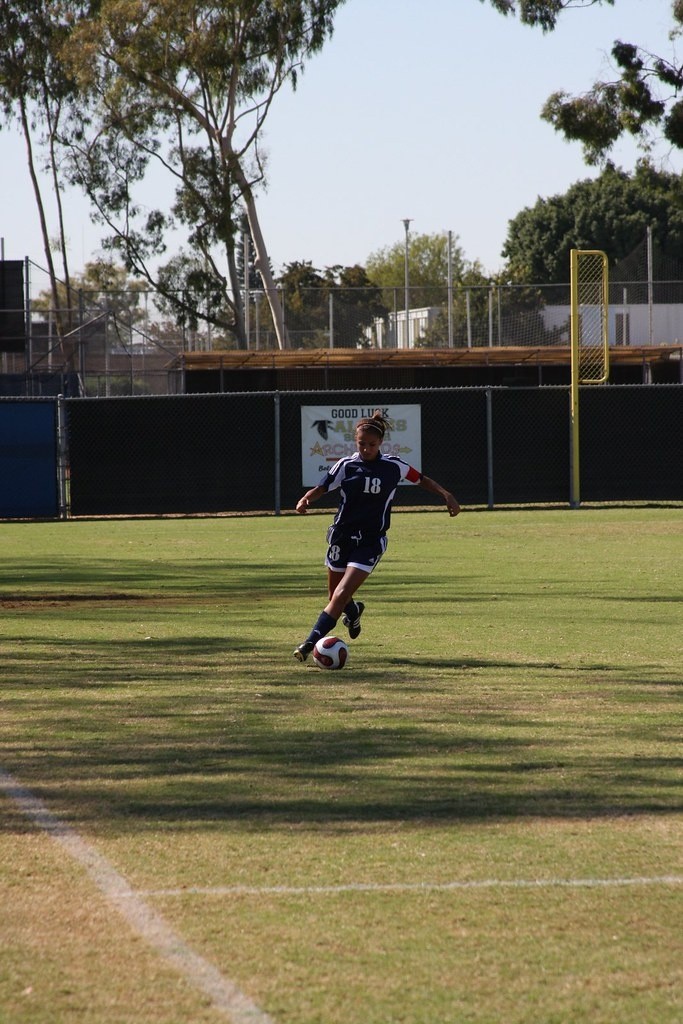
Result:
[293,410,461,663]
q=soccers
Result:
[312,635,348,671]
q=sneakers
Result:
[293,642,310,662]
[342,602,366,639]
